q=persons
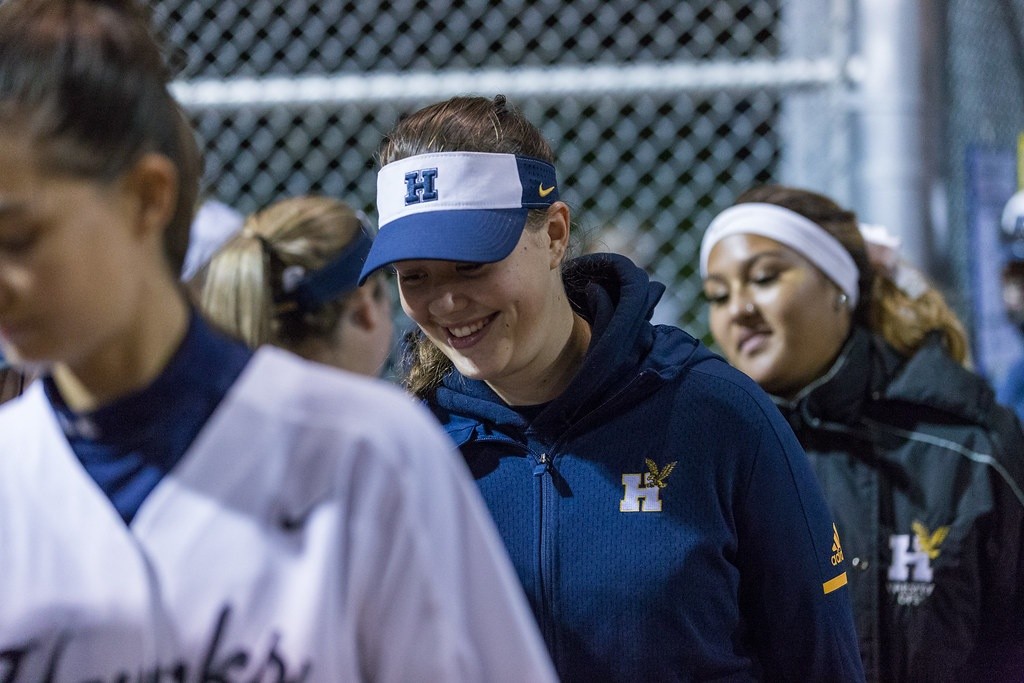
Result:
[0,0,563,683]
[175,91,1024,683]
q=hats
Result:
[356,152,560,289]
[278,209,394,316]
